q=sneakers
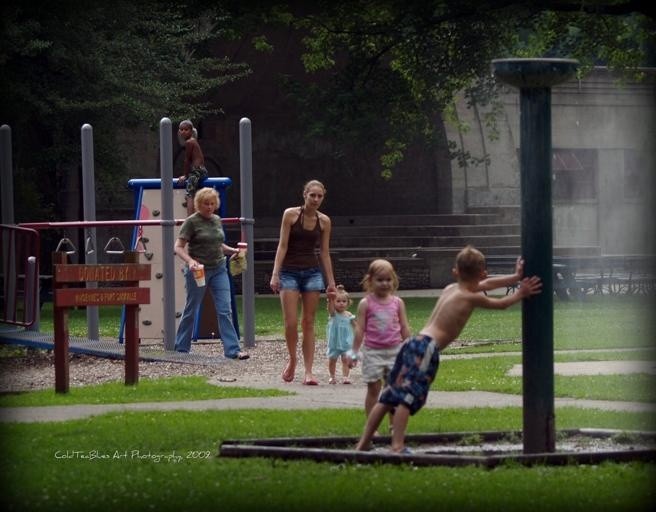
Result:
[328,377,350,384]
[227,350,250,360]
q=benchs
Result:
[483,253,656,296]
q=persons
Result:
[174,188,250,360]
[176,120,208,218]
[324,285,358,384]
[354,244,544,455]
[347,258,411,437]
[268,178,337,386]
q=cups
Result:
[237,243,247,258]
[192,264,205,288]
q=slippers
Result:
[282,363,318,385]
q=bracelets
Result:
[351,351,360,360]
